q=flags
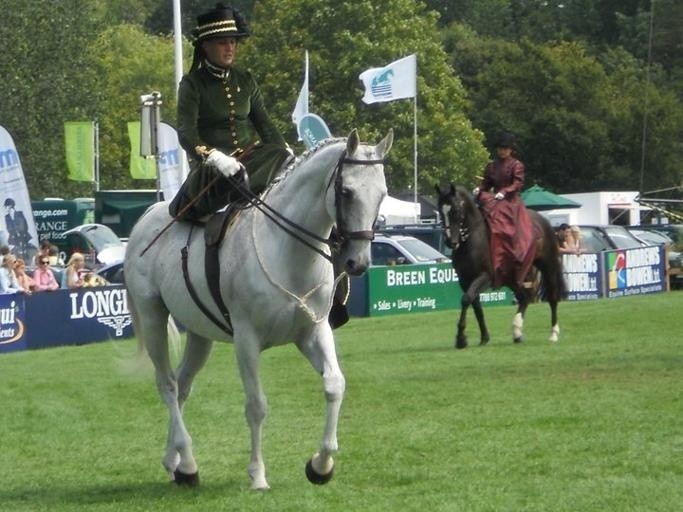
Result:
[357,53,416,106]
[290,79,308,142]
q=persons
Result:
[167,1,349,332]
[568,224,588,255]
[3,198,32,246]
[554,223,577,253]
[0,242,110,299]
[471,132,537,291]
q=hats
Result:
[3,198,15,206]
[184,9,250,46]
[492,132,519,153]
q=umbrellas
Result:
[520,183,582,211]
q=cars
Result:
[371,232,452,267]
[554,224,683,289]
[62,223,125,286]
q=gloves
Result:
[472,186,480,197]
[204,150,241,179]
[493,192,505,200]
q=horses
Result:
[123,127,394,494]
[434,181,569,349]
